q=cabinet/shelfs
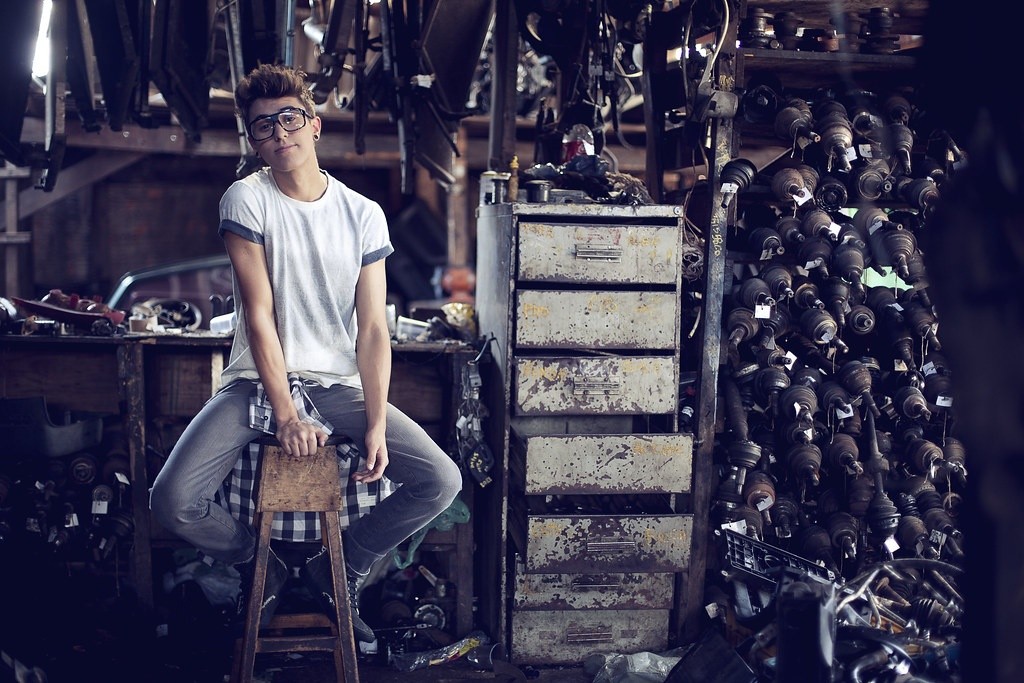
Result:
[474,204,694,667]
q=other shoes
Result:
[299,549,376,643]
[233,556,287,635]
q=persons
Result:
[148,66,461,655]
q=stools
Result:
[229,435,360,683]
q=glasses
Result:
[249,107,313,142]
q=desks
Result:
[0,336,478,641]
